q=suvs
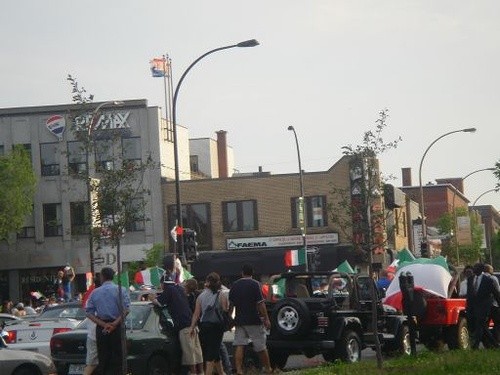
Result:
[266,270,412,370]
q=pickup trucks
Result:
[417,272,500,354]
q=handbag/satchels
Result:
[198,292,235,336]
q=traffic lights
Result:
[420,242,430,259]
[183,228,200,264]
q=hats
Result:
[161,275,175,284]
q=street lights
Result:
[418,126,478,258]
[470,188,500,263]
[341,144,374,280]
[87,101,125,284]
[287,125,309,272]
[172,39,261,270]
[453,167,497,284]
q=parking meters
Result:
[399,271,418,360]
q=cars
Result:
[0,285,238,375]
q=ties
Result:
[473,277,478,294]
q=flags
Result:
[134,266,161,287]
[329,259,355,272]
[176,258,194,283]
[171,226,183,243]
[284,249,306,266]
[387,247,417,267]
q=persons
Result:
[0,258,500,375]
